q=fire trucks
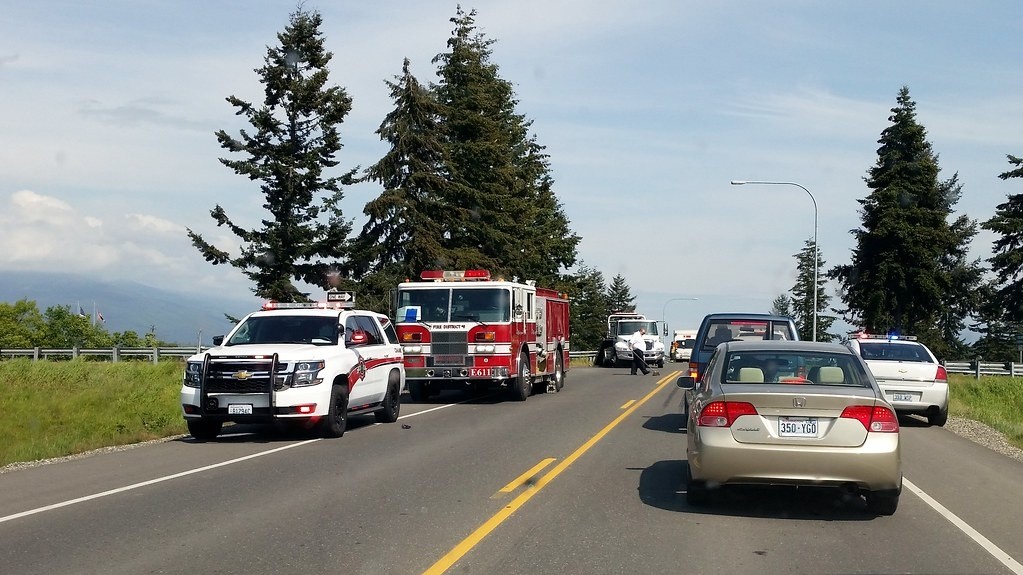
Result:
[389,269,570,401]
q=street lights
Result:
[731,180,818,342]
[662,298,699,344]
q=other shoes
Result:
[631,372,637,375]
[644,371,650,375]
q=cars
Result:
[676,340,902,516]
[830,334,949,427]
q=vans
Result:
[685,313,807,429]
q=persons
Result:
[629,327,650,375]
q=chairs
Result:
[816,367,845,385]
[738,368,764,383]
[714,328,732,341]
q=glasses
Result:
[642,331,645,334]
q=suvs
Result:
[181,299,406,439]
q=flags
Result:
[97,312,106,323]
[80,307,85,318]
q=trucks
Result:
[602,312,668,368]
[673,330,698,362]
